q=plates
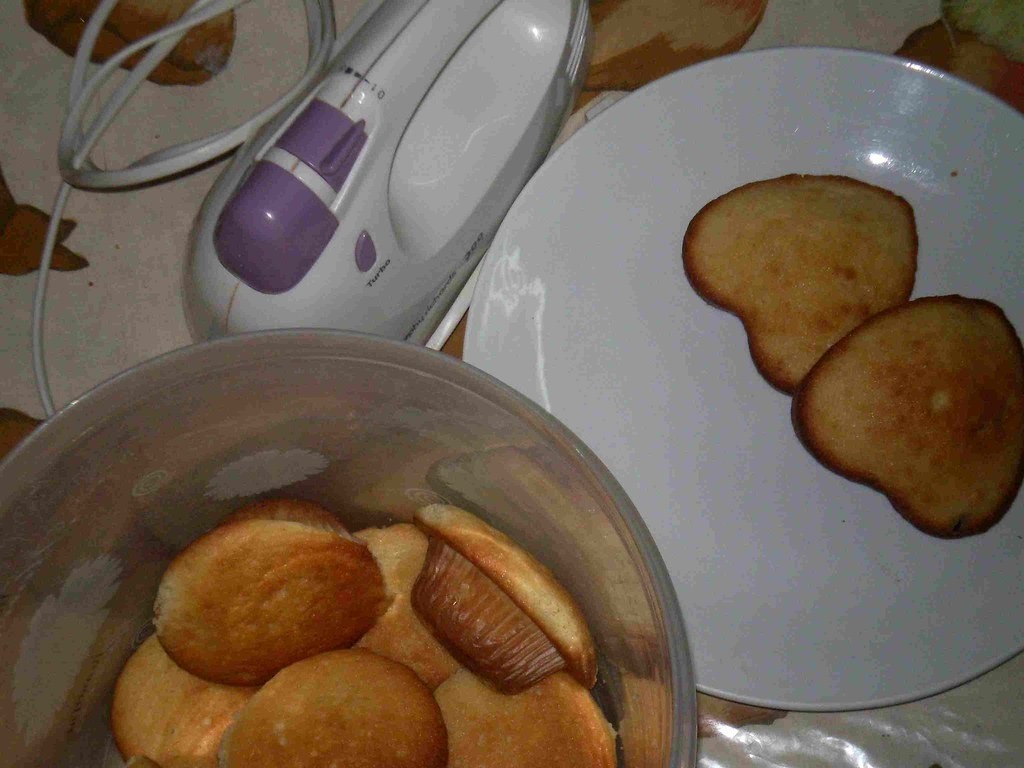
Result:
[462,48,1024,710]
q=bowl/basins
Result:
[1,330,701,768]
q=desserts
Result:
[115,500,623,768]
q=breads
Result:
[683,172,918,393]
[791,293,1024,538]
